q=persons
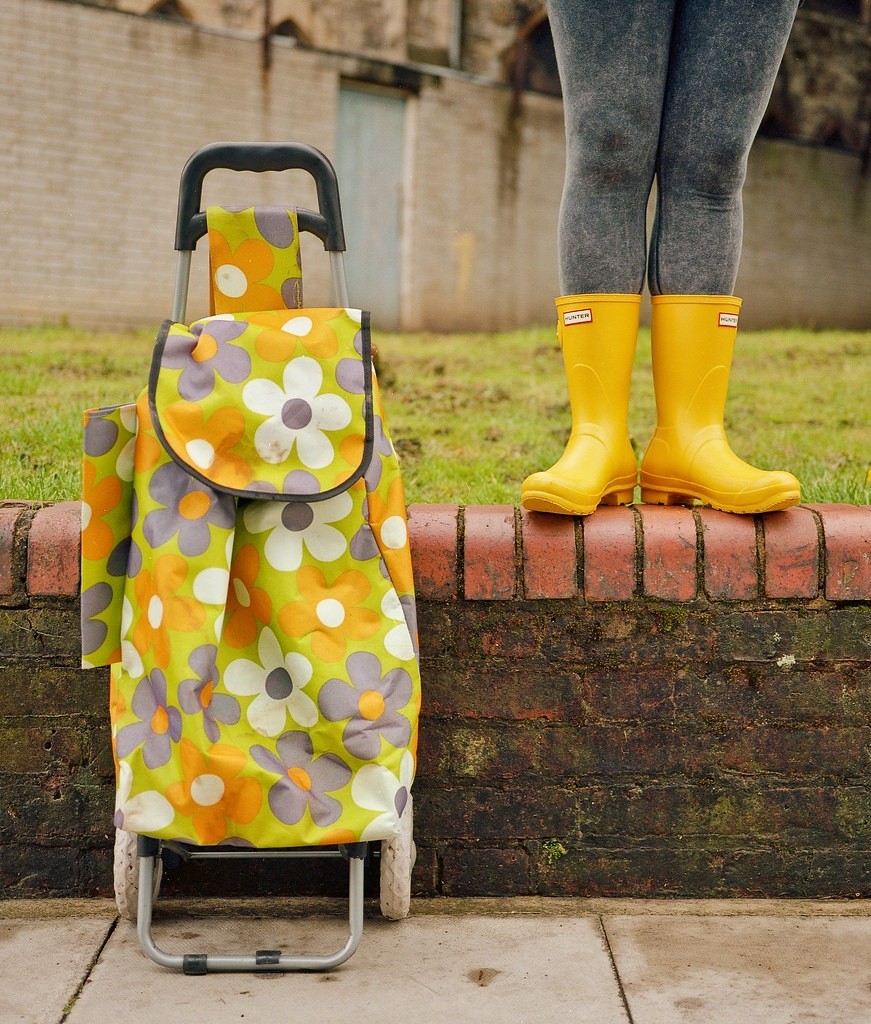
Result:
[518,0,802,516]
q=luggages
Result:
[79,142,424,973]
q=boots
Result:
[639,295,801,514]
[520,294,642,516]
